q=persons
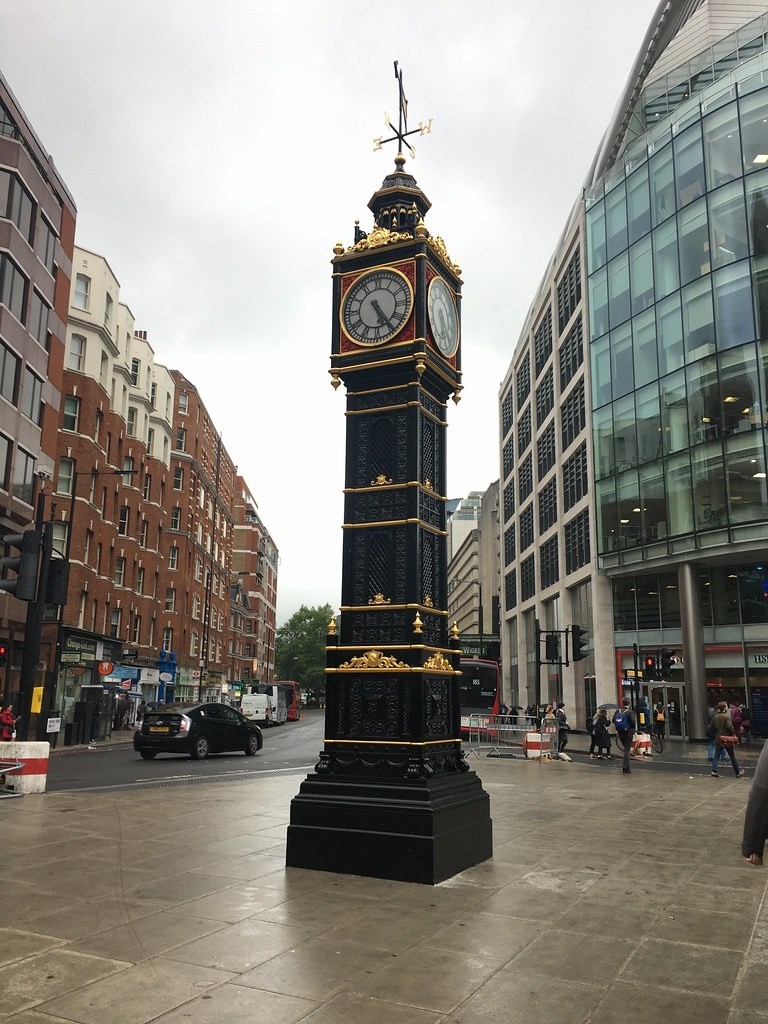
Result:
[509,708,518,733]
[1,704,16,741]
[590,709,612,759]
[612,699,637,774]
[158,699,165,705]
[729,703,752,746]
[524,705,537,726]
[741,739,768,866]
[545,705,555,718]
[706,702,745,778]
[654,702,666,739]
[707,705,729,762]
[500,704,508,718]
[136,700,147,721]
[639,703,650,731]
[556,702,571,753]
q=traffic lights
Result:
[0,645,8,667]
[571,624,590,663]
[645,658,656,677]
[661,648,675,678]
[0,529,41,603]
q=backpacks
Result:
[655,708,664,721]
[615,709,629,730]
[586,716,600,732]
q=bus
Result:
[274,681,302,723]
[459,657,500,738]
[247,683,288,726]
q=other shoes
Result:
[623,766,631,773]
[708,758,714,763]
[643,752,647,756]
[722,756,730,762]
[608,756,613,760]
[736,769,744,778]
[590,754,597,759]
[711,772,723,778]
[647,753,653,756]
[598,756,605,760]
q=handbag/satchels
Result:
[8,726,15,734]
[719,735,738,747]
[741,719,749,727]
[739,725,745,735]
[136,714,142,721]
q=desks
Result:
[693,424,718,443]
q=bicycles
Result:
[615,720,663,754]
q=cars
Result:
[133,701,263,760]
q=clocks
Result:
[426,276,459,359]
[338,267,414,348]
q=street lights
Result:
[45,470,140,741]
[198,571,251,701]
[266,629,290,682]
[452,578,483,658]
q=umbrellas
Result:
[147,701,158,707]
[510,706,523,710]
[597,704,618,719]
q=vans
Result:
[240,694,276,728]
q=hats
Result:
[643,703,648,707]
[557,702,564,709]
